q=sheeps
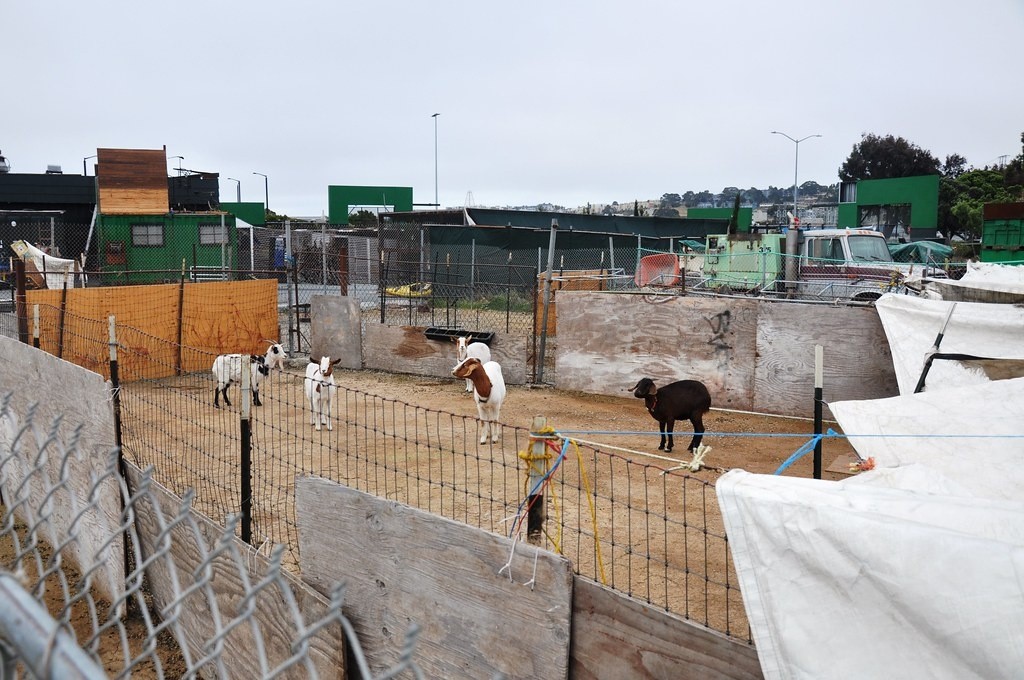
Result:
[452,356,507,445]
[450,334,492,393]
[211,339,288,409]
[627,377,711,451]
[304,355,341,431]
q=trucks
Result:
[702,226,949,308]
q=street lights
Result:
[227,177,241,202]
[253,172,269,214]
[431,110,442,210]
[771,130,822,218]
